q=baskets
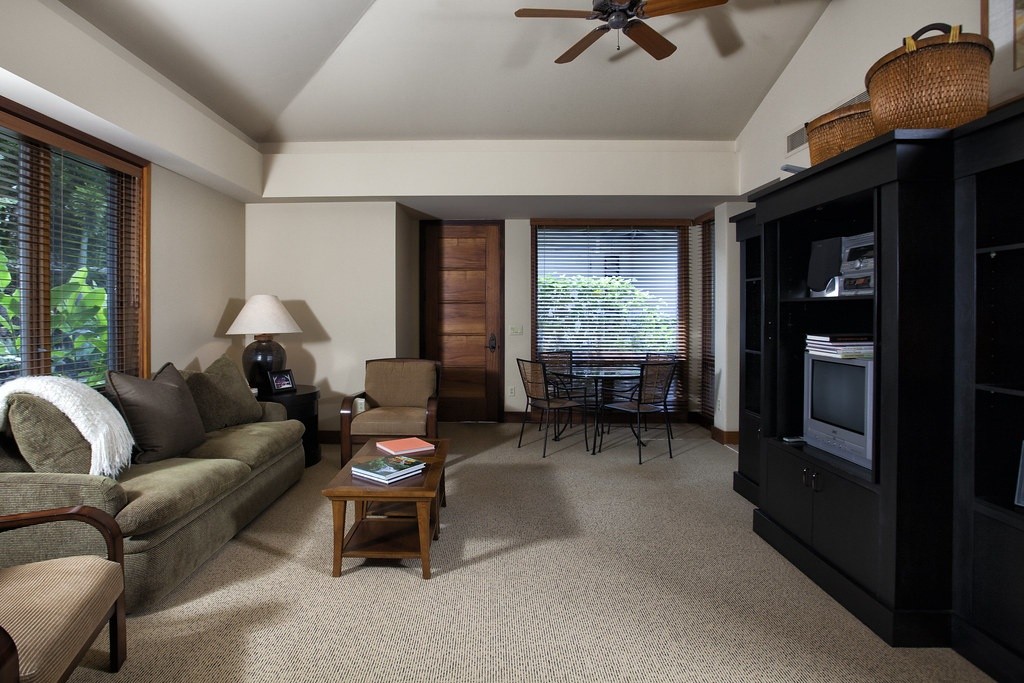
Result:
[863,22,995,138]
[804,101,875,167]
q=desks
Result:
[548,367,656,456]
[321,438,451,579]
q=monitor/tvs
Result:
[803,353,873,468]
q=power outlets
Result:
[507,386,515,398]
[717,400,720,412]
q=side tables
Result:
[248,385,322,468]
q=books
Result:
[351,455,426,484]
[804,334,873,359]
[376,437,435,455]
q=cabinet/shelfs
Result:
[733,94,1024,683]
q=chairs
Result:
[341,358,443,469]
[516,351,678,465]
[0,504,127,683]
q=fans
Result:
[514,0,729,64]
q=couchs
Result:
[0,371,304,617]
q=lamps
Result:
[225,294,303,400]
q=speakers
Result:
[807,237,842,297]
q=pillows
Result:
[8,393,92,474]
[187,355,263,432]
[104,362,207,465]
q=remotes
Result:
[783,436,803,442]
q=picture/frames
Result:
[268,368,297,395]
